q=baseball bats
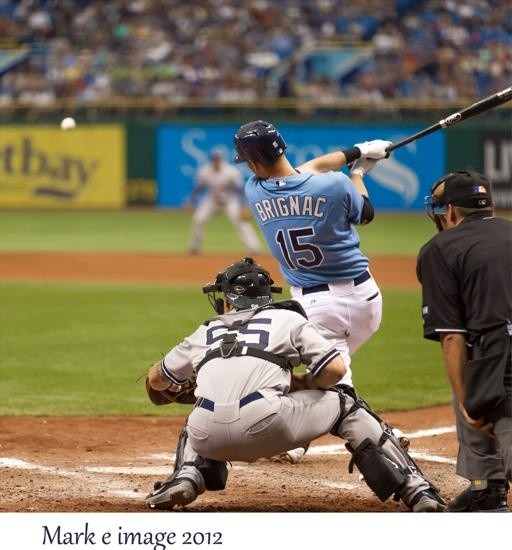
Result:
[366,86,512,161]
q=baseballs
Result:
[61,117,76,131]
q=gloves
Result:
[349,158,379,177]
[352,139,392,160]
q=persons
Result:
[145,120,512,511]
[0,0,512,108]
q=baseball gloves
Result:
[146,377,197,405]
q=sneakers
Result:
[144,476,199,511]
[358,427,410,482]
[447,478,509,512]
[267,446,306,464]
[409,487,449,512]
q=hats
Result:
[234,120,287,165]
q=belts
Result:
[194,391,264,412]
[302,271,371,295]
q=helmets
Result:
[201,256,282,316]
[424,169,494,233]
